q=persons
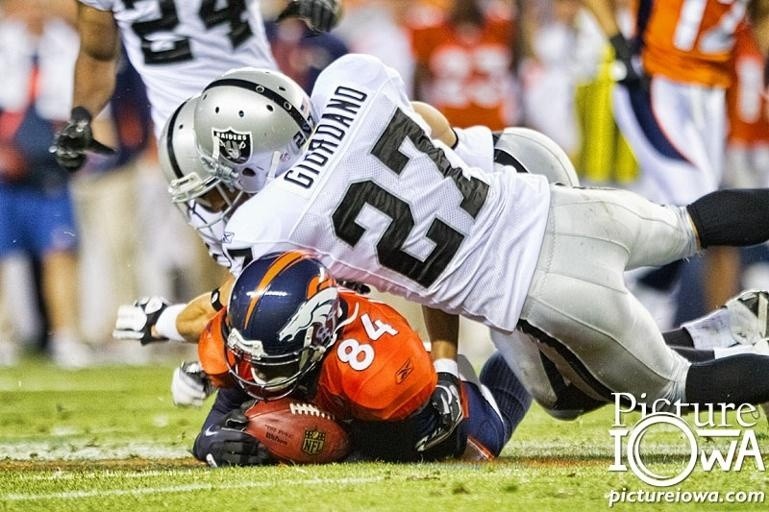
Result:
[1,1,769,380]
[110,51,769,416]
[162,92,769,421]
[192,249,538,471]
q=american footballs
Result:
[243,399,352,466]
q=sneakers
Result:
[719,291,769,346]
[41,330,91,371]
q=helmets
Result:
[220,251,360,403]
[193,67,320,195]
[156,96,254,245]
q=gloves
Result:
[273,0,341,34]
[169,360,218,408]
[413,372,466,453]
[55,106,116,175]
[111,295,172,346]
[199,408,270,469]
